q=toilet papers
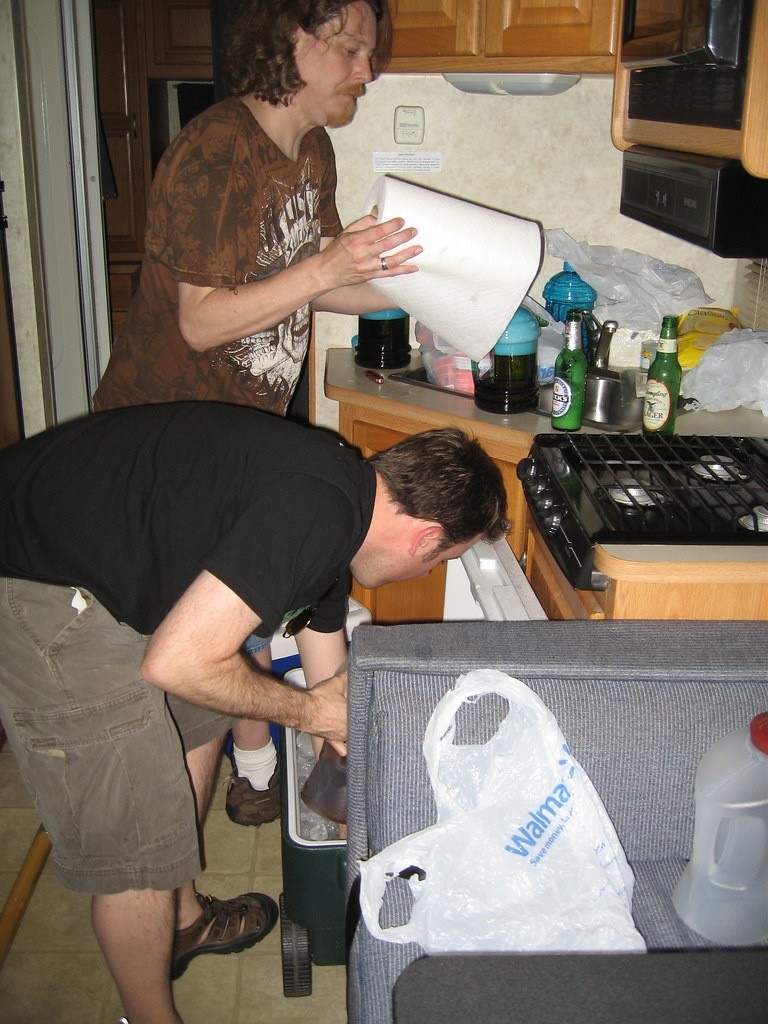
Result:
[361,176,541,362]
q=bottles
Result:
[642,316,682,438]
[550,309,586,433]
[541,259,597,350]
[355,307,412,370]
[468,301,541,415]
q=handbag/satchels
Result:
[357,668,648,959]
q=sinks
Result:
[528,367,698,433]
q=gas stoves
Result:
[516,433,768,591]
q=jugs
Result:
[671,713,768,946]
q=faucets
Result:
[581,310,617,368]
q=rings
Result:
[381,258,389,271]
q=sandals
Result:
[169,892,279,979]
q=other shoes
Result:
[221,755,281,827]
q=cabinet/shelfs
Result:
[369,0,622,73]
[324,344,768,626]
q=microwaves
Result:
[622,0,750,71]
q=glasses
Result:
[282,576,339,638]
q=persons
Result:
[0,400,509,1024]
[91,0,423,828]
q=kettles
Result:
[584,319,653,433]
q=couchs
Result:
[348,620,768,1023]
